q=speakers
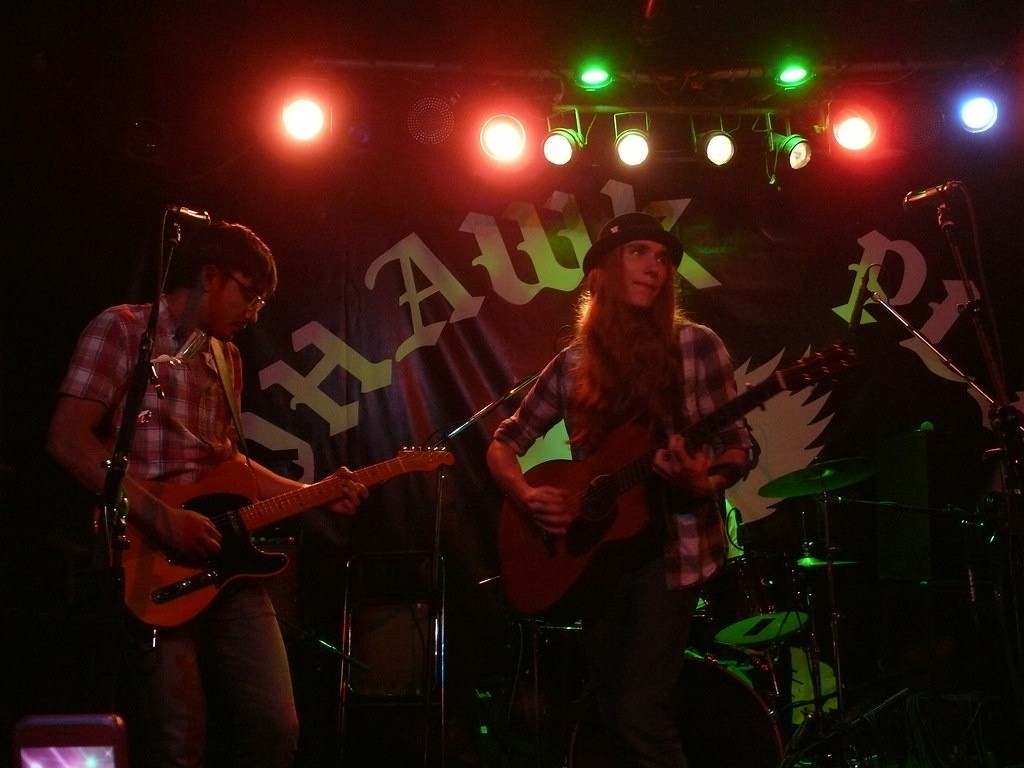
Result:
[342,552,436,703]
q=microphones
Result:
[735,510,752,545]
[848,269,872,331]
[903,180,962,214]
[168,206,210,231]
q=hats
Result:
[583,213,684,272]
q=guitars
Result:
[83,446,455,632]
[498,341,874,620]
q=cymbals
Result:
[758,456,880,498]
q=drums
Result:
[567,649,786,768]
[713,610,813,677]
[345,590,447,708]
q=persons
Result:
[488,214,756,768]
[49,222,368,768]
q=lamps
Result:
[614,112,652,166]
[752,112,812,185]
[771,66,816,94]
[541,108,597,166]
[689,112,743,166]
[574,69,615,94]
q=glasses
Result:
[224,272,265,311]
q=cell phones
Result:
[10,713,128,768]
